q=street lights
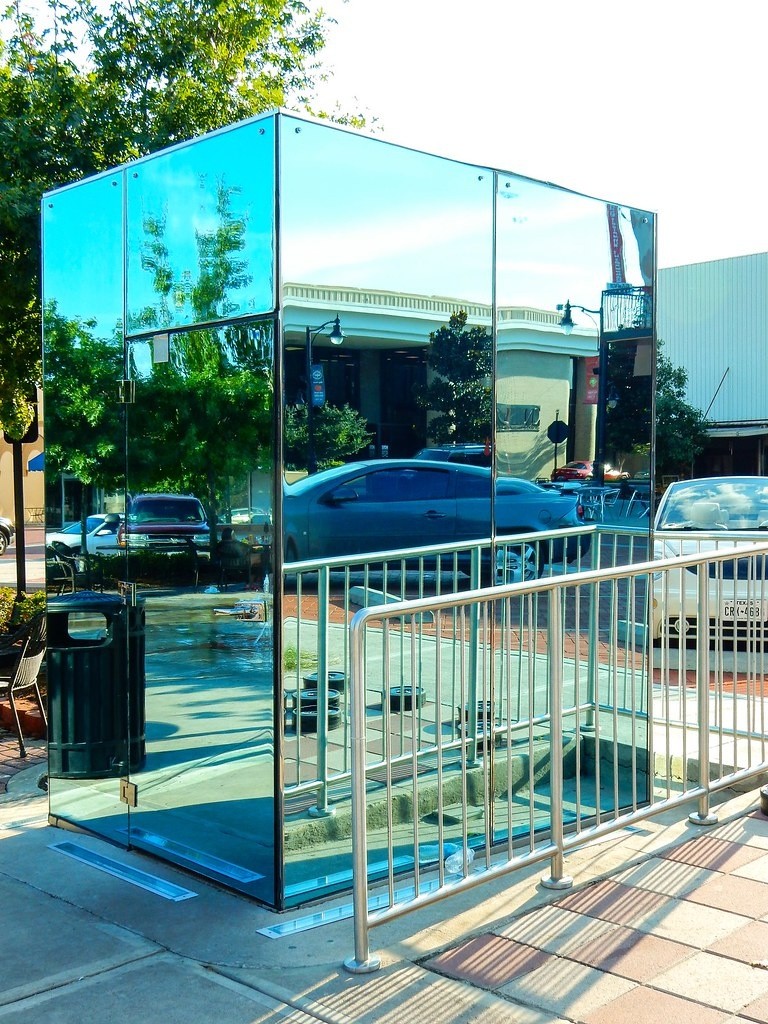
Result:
[556,299,605,487]
[306,313,348,476]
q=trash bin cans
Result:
[45,589,149,779]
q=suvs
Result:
[414,441,492,469]
[128,492,211,570]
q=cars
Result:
[650,475,768,648]
[551,460,620,483]
[218,506,265,523]
[276,458,593,591]
[45,512,126,569]
[0,517,16,556]
